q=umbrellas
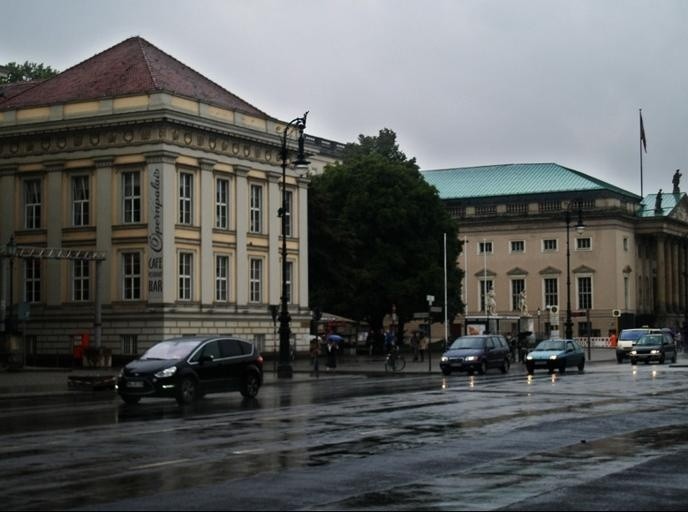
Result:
[326,334,343,341]
[306,334,321,341]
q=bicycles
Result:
[383,345,406,377]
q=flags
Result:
[640,112,649,154]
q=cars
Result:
[631,333,677,364]
[115,334,264,406]
[356,331,393,357]
[439,334,585,375]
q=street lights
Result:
[6,234,16,331]
[277,110,312,379]
[537,307,541,338]
[563,198,588,339]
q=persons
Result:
[323,338,339,372]
[411,334,418,362]
[306,341,323,376]
[506,333,533,364]
[606,330,617,349]
[486,286,499,318]
[517,289,534,316]
[655,188,664,216]
[671,168,682,193]
[675,331,682,353]
[418,336,429,363]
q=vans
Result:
[615,328,661,363]
